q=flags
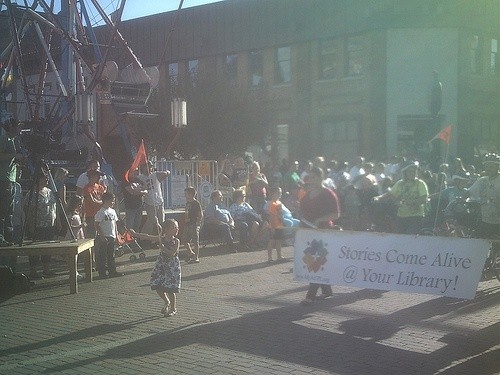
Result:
[124,145,145,182]
[427,126,451,145]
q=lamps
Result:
[120,112,161,119]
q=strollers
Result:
[113,208,146,264]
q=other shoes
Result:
[164,310,177,317]
[185,251,195,262]
[110,272,123,277]
[301,298,313,306]
[195,259,200,263]
[161,302,170,315]
[99,274,108,279]
[317,293,333,300]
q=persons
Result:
[263,158,302,208]
[180,187,203,263]
[53,168,70,198]
[268,186,285,263]
[221,152,269,248]
[371,161,429,235]
[465,153,500,238]
[94,193,118,278]
[205,190,238,250]
[387,155,480,237]
[66,195,87,281]
[300,167,340,309]
[303,153,392,232]
[21,171,56,278]
[76,158,108,271]
[120,160,170,242]
[127,219,181,316]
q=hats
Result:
[401,160,418,172]
[481,152,500,165]
[86,170,106,177]
[142,160,154,168]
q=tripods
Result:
[19,160,78,247]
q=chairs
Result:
[199,210,223,249]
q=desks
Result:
[0,237,95,294]
